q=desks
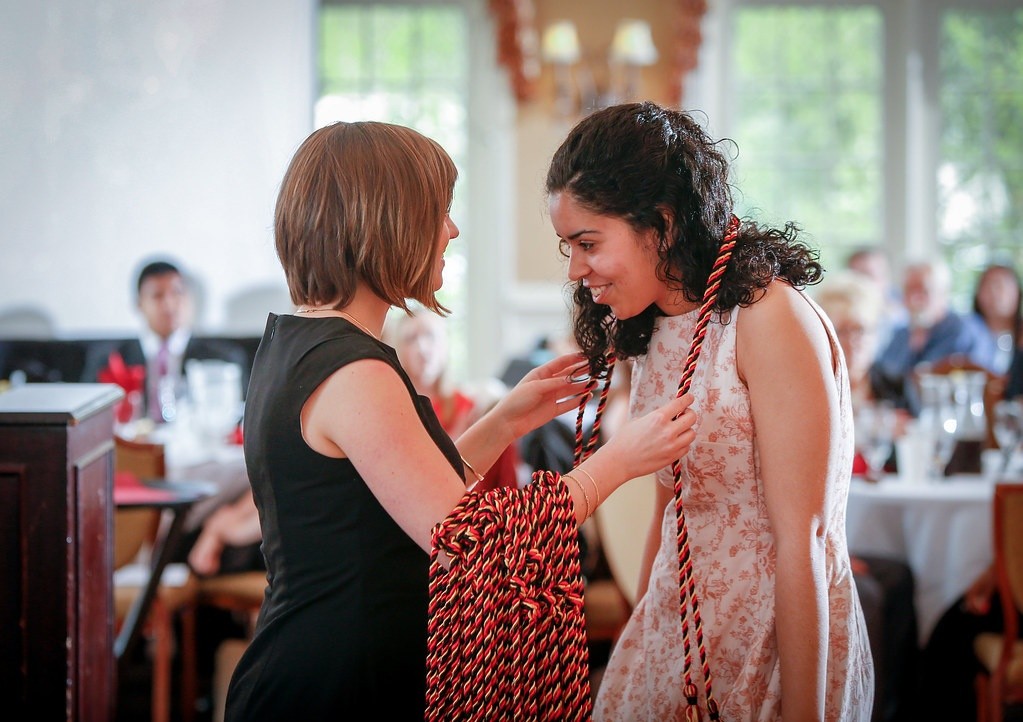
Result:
[848,472,1023,646]
[110,476,218,667]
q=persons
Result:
[96,244,1023,573]
[221,121,697,722]
[542,102,876,722]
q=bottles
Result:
[952,369,988,445]
[896,374,957,483]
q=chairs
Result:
[972,482,1023,722]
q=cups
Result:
[993,402,1023,487]
[856,402,896,473]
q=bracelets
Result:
[460,455,484,481]
[560,475,590,524]
[574,468,599,513]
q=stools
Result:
[148,572,278,721]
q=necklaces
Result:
[292,307,385,344]
